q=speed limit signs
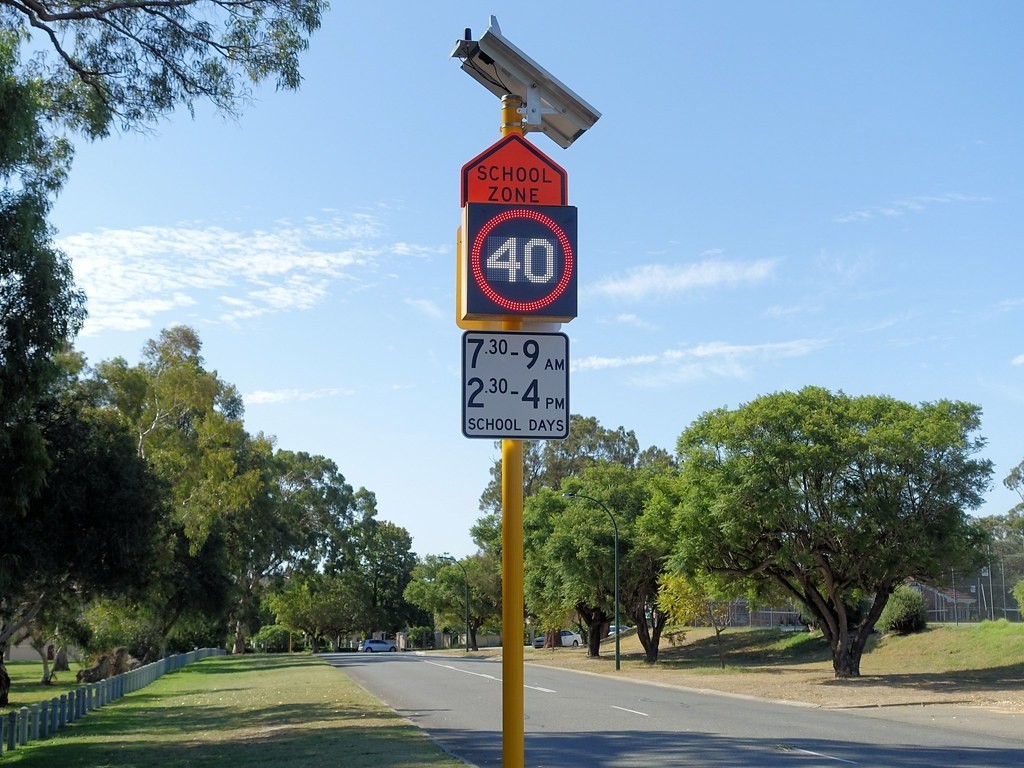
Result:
[459,201,577,321]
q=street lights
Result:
[437,556,469,652]
[562,493,620,671]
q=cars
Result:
[532,629,582,648]
[358,640,397,652]
[609,626,630,636]
